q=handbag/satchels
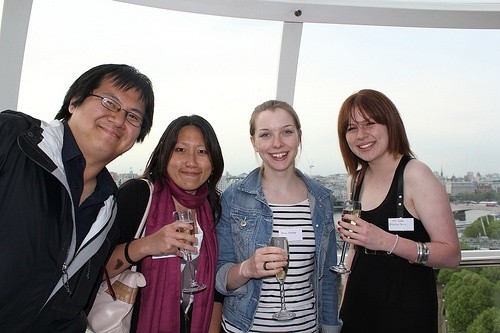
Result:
[84,179,154,333]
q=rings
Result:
[263,261,269,271]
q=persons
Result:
[215,100,343,333]
[102,114,225,333]
[0,64,154,333]
[337,89,461,333]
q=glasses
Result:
[90,94,145,129]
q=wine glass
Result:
[270,236,296,321]
[172,209,206,293]
[329,200,362,273]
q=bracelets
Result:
[409,242,429,265]
[387,234,398,254]
[124,238,143,265]
